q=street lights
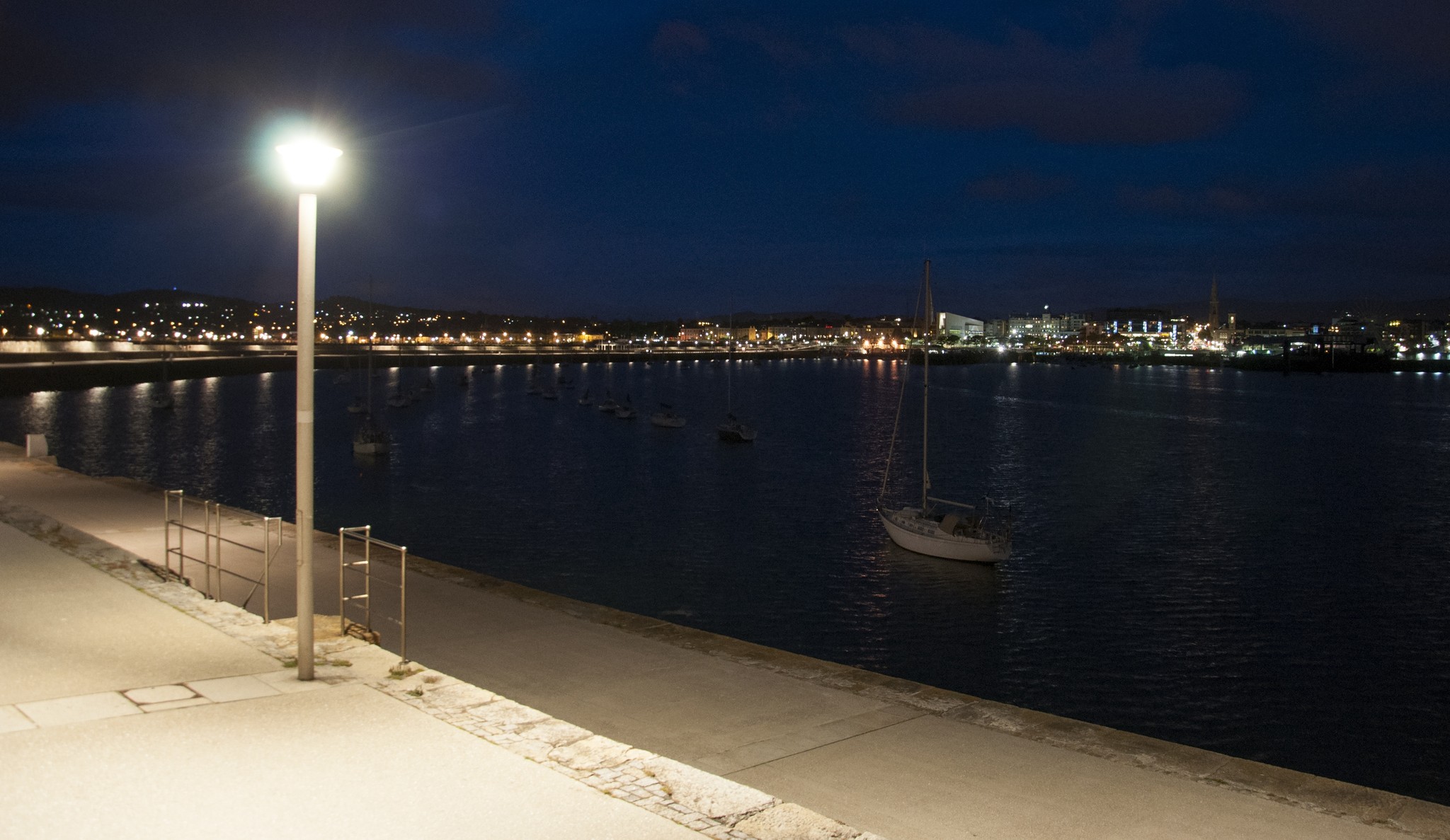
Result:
[271,123,352,685]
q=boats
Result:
[351,370,759,468]
[875,258,1014,564]
